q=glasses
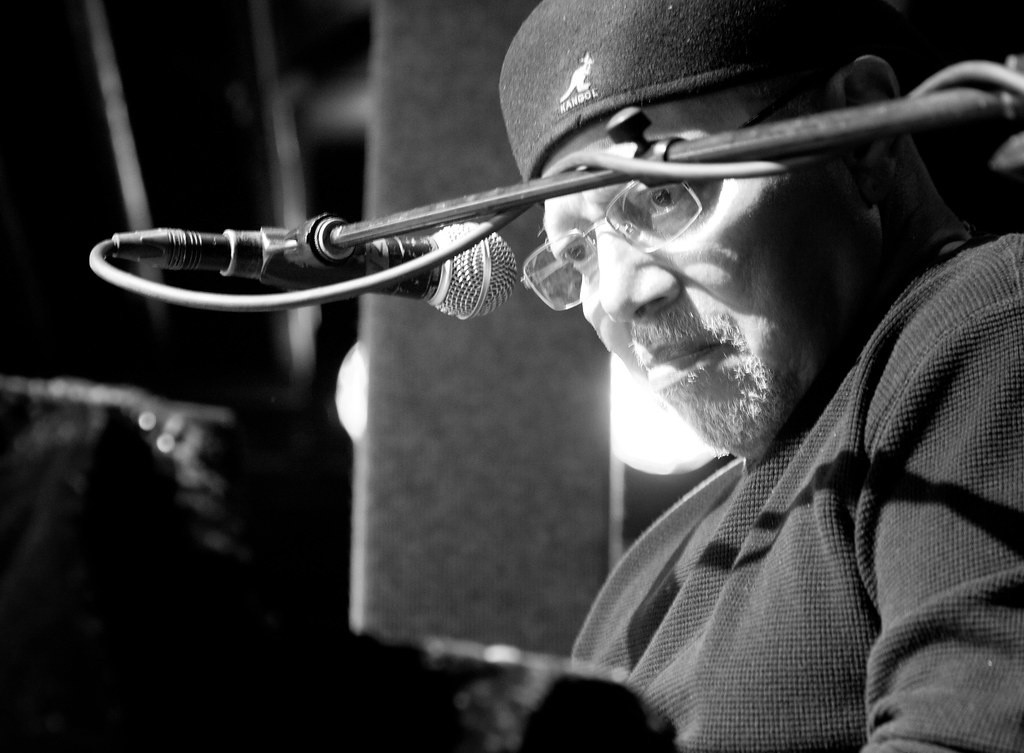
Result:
[521,77,825,311]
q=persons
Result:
[497,0,1024,753]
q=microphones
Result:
[112,221,516,320]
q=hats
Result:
[499,0,906,182]
